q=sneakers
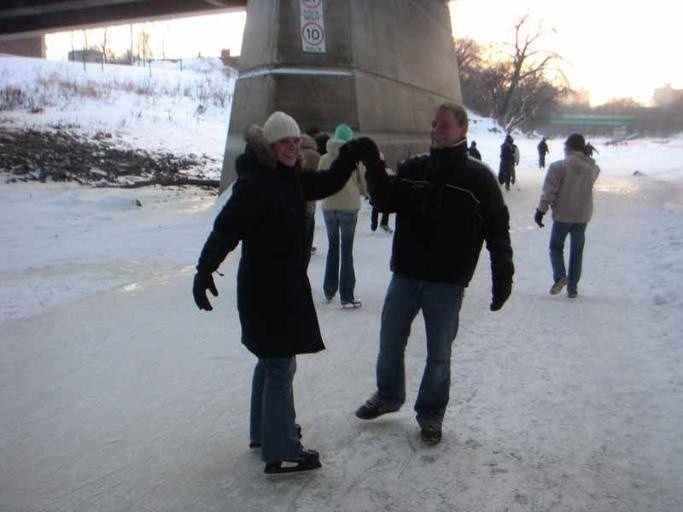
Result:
[550,277,567,294]
[341,297,361,304]
[416,414,441,444]
[251,424,301,447]
[356,394,401,418]
[569,291,576,298]
[325,288,335,298]
[265,445,319,461]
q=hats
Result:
[264,111,300,144]
[565,134,584,151]
[335,124,352,140]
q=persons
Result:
[585,142,597,157]
[193,112,359,473]
[317,125,370,305]
[300,127,316,254]
[357,102,514,446]
[468,141,481,161]
[372,152,392,231]
[499,134,519,191]
[535,134,600,298]
[538,137,549,168]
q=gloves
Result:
[194,269,218,310]
[490,288,509,311]
[534,209,544,226]
[341,138,379,166]
[584,144,592,155]
